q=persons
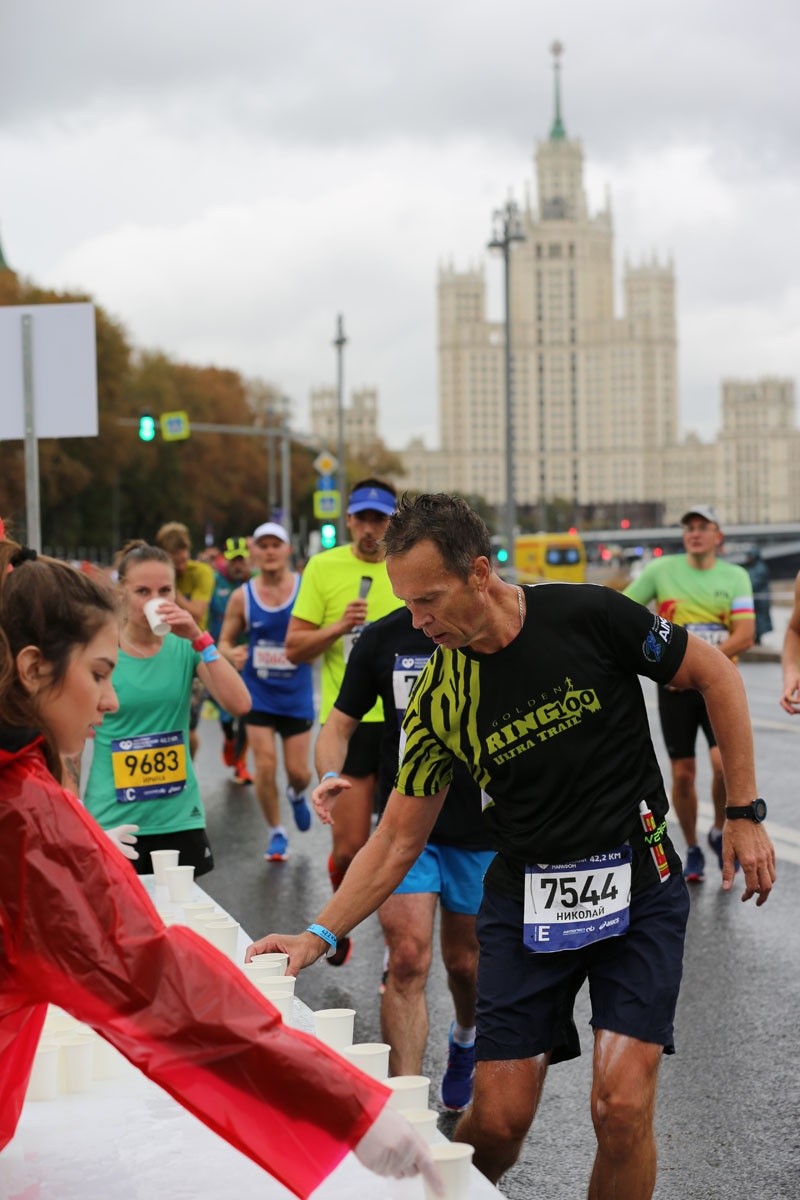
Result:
[779,572,800,715]
[245,491,777,1200]
[621,503,755,883]
[745,544,774,646]
[0,477,498,1115]
[0,540,444,1199]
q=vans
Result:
[512,532,588,584]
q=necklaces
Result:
[516,585,525,631]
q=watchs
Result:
[724,799,767,824]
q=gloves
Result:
[103,824,140,859]
[354,1104,447,1198]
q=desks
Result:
[0,876,505,1200]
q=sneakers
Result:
[439,1019,476,1112]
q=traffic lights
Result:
[319,522,337,549]
[139,409,156,443]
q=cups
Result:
[241,962,281,985]
[262,991,293,1025]
[196,913,228,940]
[425,1142,476,1200]
[205,922,240,961]
[313,1008,356,1051]
[343,1043,392,1083]
[143,597,172,637]
[399,1109,440,1146]
[251,953,289,976]
[150,850,180,886]
[164,865,196,903]
[255,976,296,1002]
[383,1075,431,1109]
[183,903,215,930]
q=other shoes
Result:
[222,730,237,767]
[326,937,353,966]
[264,833,289,861]
[707,827,740,873]
[231,761,255,785]
[379,945,391,996]
[683,846,706,882]
[286,784,311,831]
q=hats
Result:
[346,487,398,516]
[223,537,252,561]
[678,504,720,529]
[254,522,291,544]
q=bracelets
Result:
[306,924,337,960]
[191,630,215,651]
[201,643,219,663]
[320,771,340,784]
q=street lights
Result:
[484,195,532,584]
[332,310,349,546]
